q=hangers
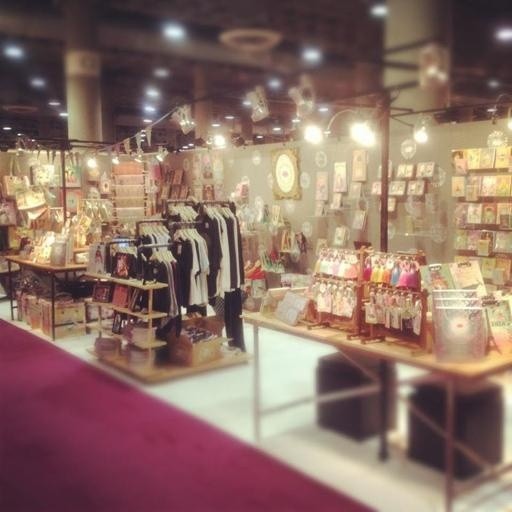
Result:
[109,196,234,268]
[309,243,422,307]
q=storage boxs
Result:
[177,309,232,366]
[15,288,85,340]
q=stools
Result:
[314,354,502,477]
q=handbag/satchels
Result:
[1,157,31,199]
[15,175,47,211]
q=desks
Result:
[6,256,87,342]
[240,307,512,512]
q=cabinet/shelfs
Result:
[112,173,148,211]
[81,271,168,372]
